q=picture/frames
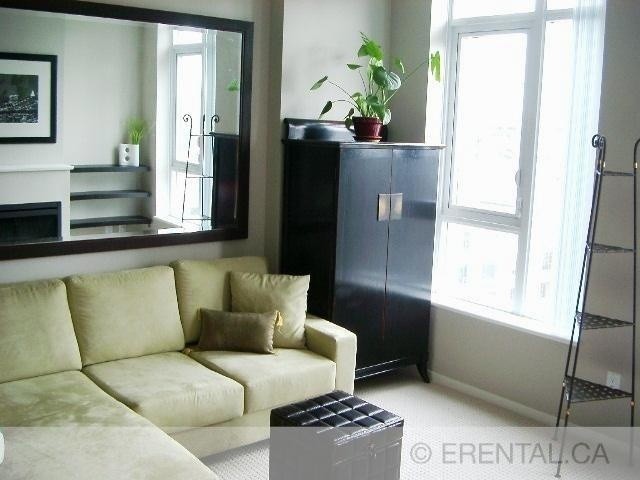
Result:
[1,53,58,143]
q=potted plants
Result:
[310,30,441,143]
[116,114,149,167]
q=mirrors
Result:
[1,0,255,260]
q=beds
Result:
[269,389,405,479]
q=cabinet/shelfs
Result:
[280,138,447,383]
[209,132,240,230]
[68,164,151,229]
[181,113,220,231]
[552,134,640,478]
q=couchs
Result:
[1,256,358,479]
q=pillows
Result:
[229,270,312,349]
[197,307,283,354]
